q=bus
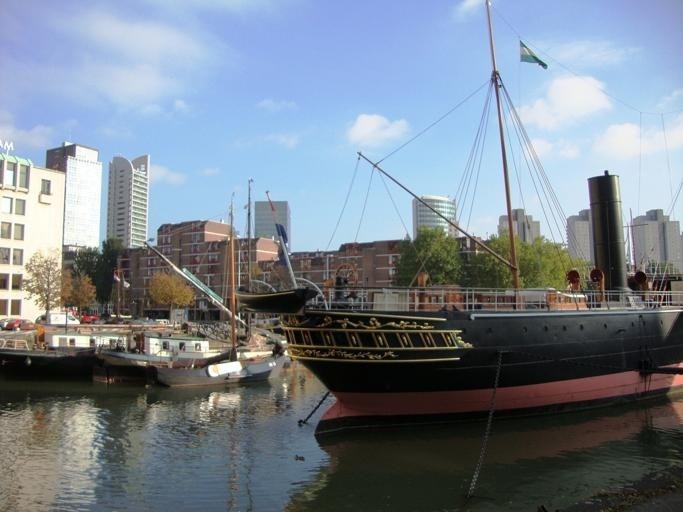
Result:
[67,309,97,323]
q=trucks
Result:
[49,312,81,325]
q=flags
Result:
[269,197,288,265]
[521,43,547,70]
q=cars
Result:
[0,318,33,330]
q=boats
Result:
[0,328,238,371]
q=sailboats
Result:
[281,0,683,442]
[234,177,319,313]
[155,192,286,387]
